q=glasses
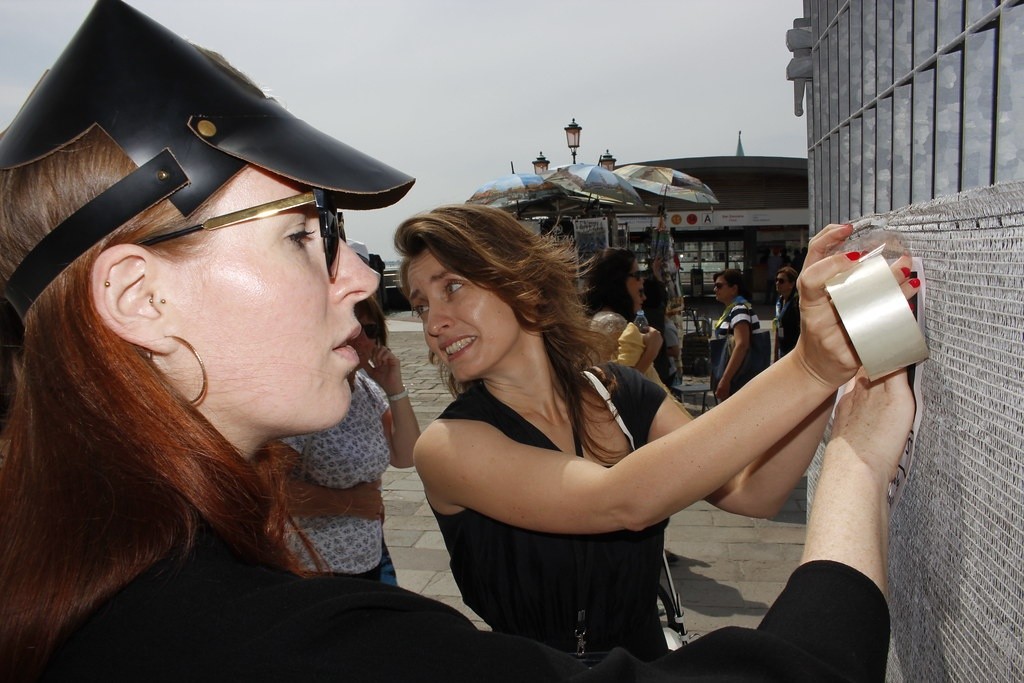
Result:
[715,282,727,289]
[362,323,380,338]
[131,186,345,284]
[627,270,641,280]
[776,278,789,284]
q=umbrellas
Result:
[612,165,719,229]
[463,162,560,221]
[538,153,648,213]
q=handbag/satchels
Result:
[711,302,771,391]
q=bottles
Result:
[633,309,650,334]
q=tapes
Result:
[823,253,930,382]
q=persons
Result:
[642,256,671,389]
[0,0,914,682]
[771,266,801,363]
[582,246,695,420]
[394,202,921,658]
[250,293,422,582]
[710,271,761,402]
[791,247,808,271]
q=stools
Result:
[668,383,718,419]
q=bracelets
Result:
[387,386,409,402]
